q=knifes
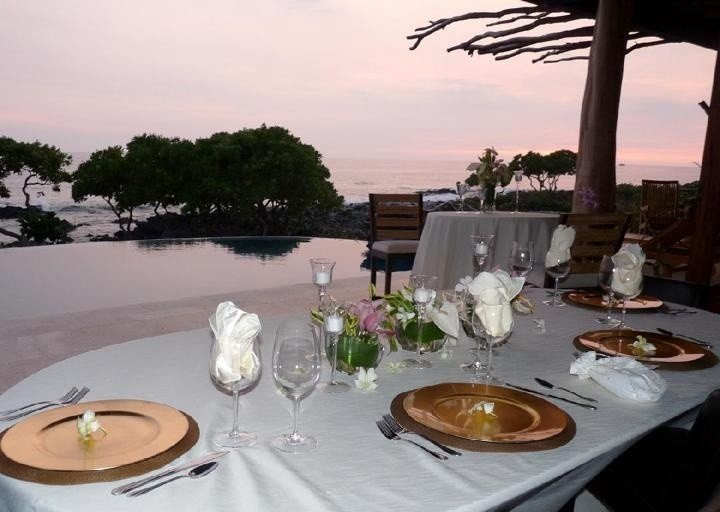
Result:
[112,449,230,494]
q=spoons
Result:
[534,377,600,404]
[128,462,219,508]
[655,327,713,347]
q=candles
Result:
[326,314,342,334]
[414,286,429,303]
[315,266,329,286]
[475,241,489,256]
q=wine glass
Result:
[467,233,496,271]
[402,274,437,370]
[271,321,321,453]
[608,268,643,330]
[596,254,622,324]
[503,240,536,274]
[513,171,524,215]
[309,258,336,309]
[321,311,351,395]
[476,189,487,215]
[459,295,495,376]
[208,337,261,449]
[475,304,515,386]
[545,249,572,307]
[457,184,466,215]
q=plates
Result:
[562,288,664,310]
[2,399,199,486]
[572,329,719,370]
[391,382,577,452]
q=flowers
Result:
[382,286,462,338]
[311,292,397,338]
[465,148,514,187]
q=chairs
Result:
[638,179,678,277]
[369,192,424,299]
[547,211,632,286]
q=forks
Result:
[382,415,464,456]
[504,383,597,410]
[0,385,90,423]
[375,419,449,461]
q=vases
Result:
[324,330,384,374]
[479,186,496,212]
[396,320,447,354]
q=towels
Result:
[208,300,261,384]
[612,243,645,295]
[469,270,525,337]
[545,224,576,268]
